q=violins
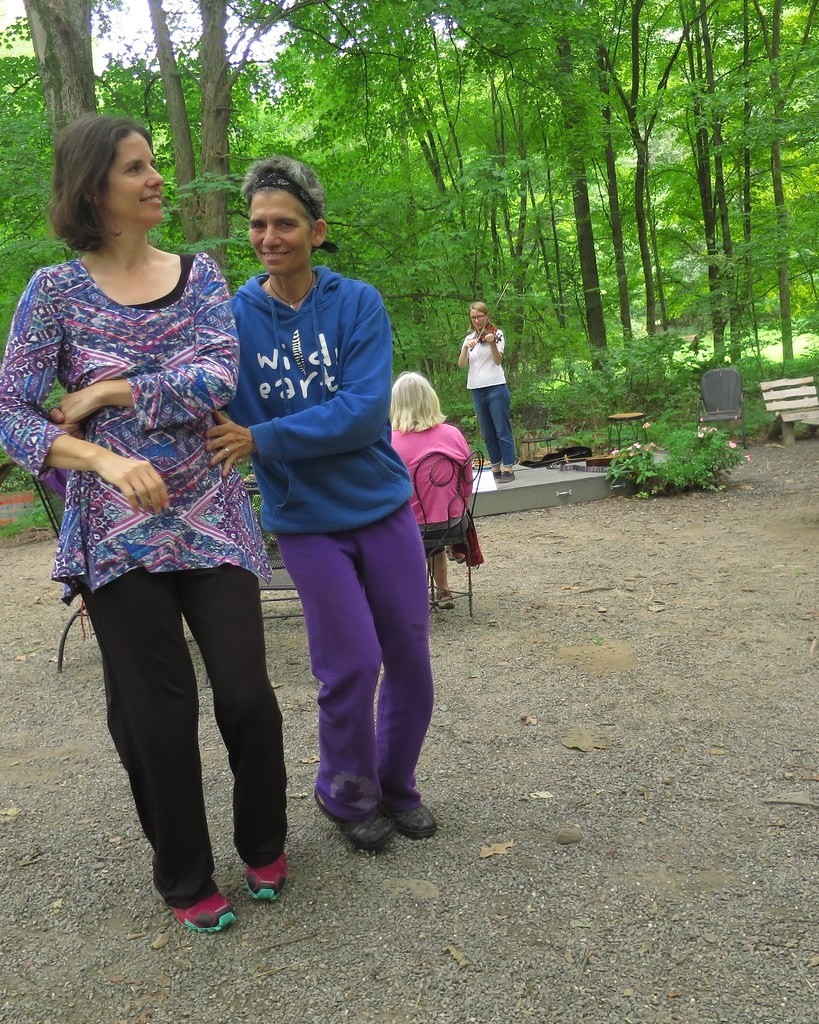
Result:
[477,323,503,344]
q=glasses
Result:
[470,315,486,321]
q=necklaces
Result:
[269,271,314,309]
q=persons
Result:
[50,156,437,849]
[0,118,289,931]
[458,302,517,483]
[391,372,472,609]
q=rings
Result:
[225,448,230,453]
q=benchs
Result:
[760,377,819,421]
[30,450,489,672]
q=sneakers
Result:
[244,850,288,901]
[171,890,236,936]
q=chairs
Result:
[605,369,652,455]
[521,405,554,461]
[696,367,748,450]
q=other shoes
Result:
[452,551,466,564]
[498,471,515,483]
[377,803,437,840]
[436,590,456,609]
[308,786,395,848]
[492,470,501,479]
